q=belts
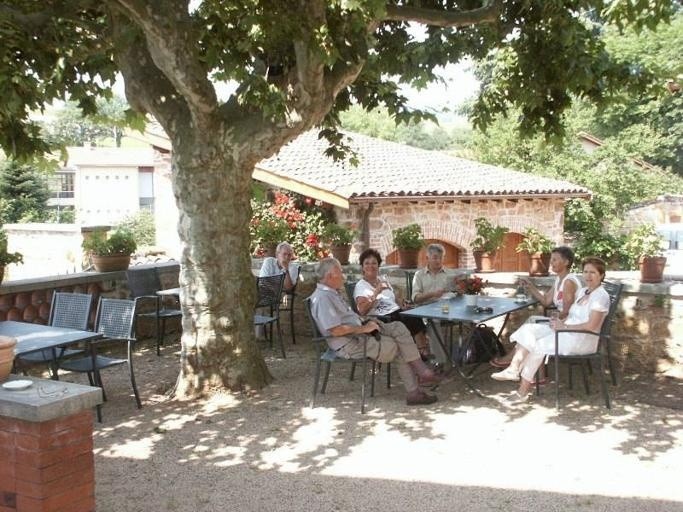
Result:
[333,340,355,353]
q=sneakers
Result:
[532,378,549,386]
[489,357,511,368]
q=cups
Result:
[440,297,450,314]
[549,310,560,331]
[378,275,390,289]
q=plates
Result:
[3,380,33,391]
[477,307,493,314]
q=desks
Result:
[156,287,181,346]
[0,321,107,402]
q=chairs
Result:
[126,266,182,355]
[19,290,94,386]
[255,273,287,358]
[264,266,301,344]
[58,296,142,423]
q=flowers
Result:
[248,189,329,262]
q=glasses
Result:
[473,306,493,316]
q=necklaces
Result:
[585,289,591,296]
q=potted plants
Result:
[391,223,425,269]
[469,216,510,272]
[514,225,556,276]
[0,230,24,285]
[624,223,669,282]
[81,224,137,272]
[326,223,356,265]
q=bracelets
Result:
[565,324,568,329]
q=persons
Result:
[310,257,451,405]
[491,257,611,405]
[254,242,298,343]
[411,243,457,371]
[489,247,582,386]
[353,248,435,361]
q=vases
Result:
[0,335,17,382]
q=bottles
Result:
[513,286,528,304]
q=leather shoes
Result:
[417,372,455,387]
[407,393,437,405]
[419,347,436,362]
[490,371,520,381]
[503,393,530,404]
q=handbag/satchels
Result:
[467,324,505,364]
